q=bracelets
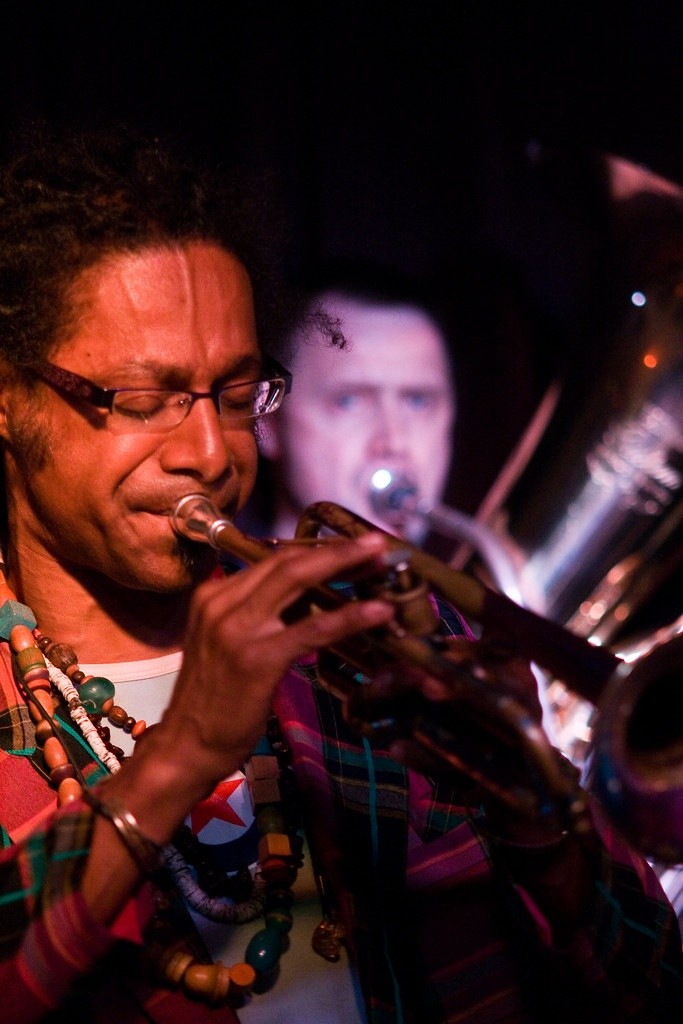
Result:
[88,783,160,871]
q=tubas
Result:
[367,149,683,751]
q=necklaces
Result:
[12,615,352,999]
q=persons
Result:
[0,124,398,1024]
[258,265,683,1024]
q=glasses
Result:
[12,352,294,433]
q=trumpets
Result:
[167,493,682,874]
